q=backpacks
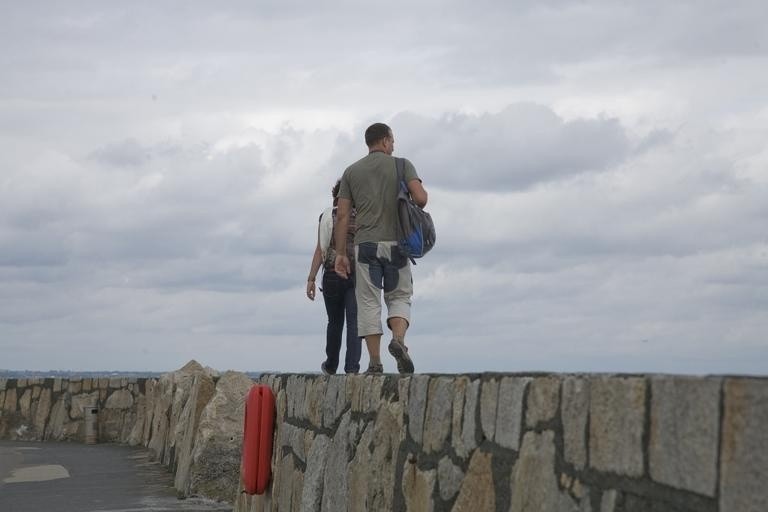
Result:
[325,205,357,275]
[395,156,436,259]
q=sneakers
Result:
[344,367,359,376]
[387,339,415,374]
[363,363,384,375]
[321,361,337,377]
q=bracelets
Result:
[307,276,315,282]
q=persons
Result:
[332,122,436,374]
[306,178,362,374]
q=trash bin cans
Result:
[83,406,98,446]
